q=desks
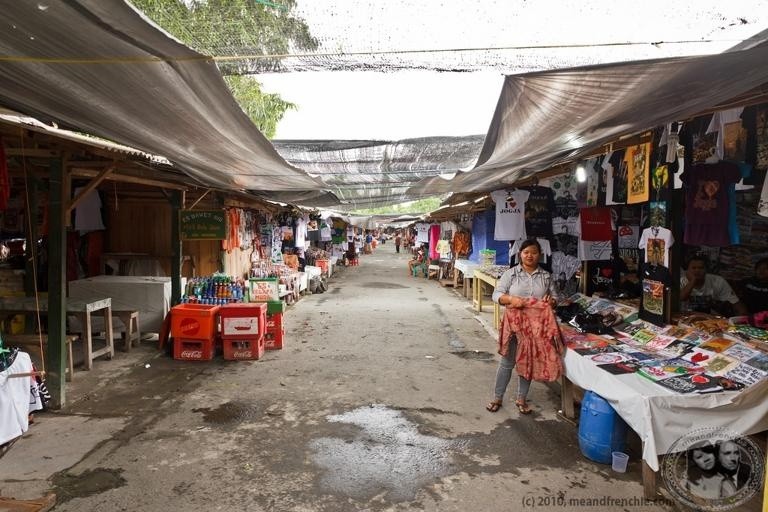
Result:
[1,296,115,371]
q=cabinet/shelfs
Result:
[454,259,478,298]
[284,272,308,300]
[68,274,188,344]
[317,254,337,278]
[473,265,508,329]
[303,264,322,291]
[548,292,767,500]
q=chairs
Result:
[414,253,427,277]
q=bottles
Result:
[234,286,237,299]
[190,296,194,305]
[218,283,222,298]
[237,341,250,349]
[180,299,185,303]
[184,298,190,303]
[237,284,241,298]
[267,333,272,338]
[242,280,245,294]
[213,274,236,283]
[214,283,219,299]
[206,299,209,304]
[228,284,232,298]
[195,298,199,304]
[209,297,213,304]
[223,300,226,304]
[214,298,218,305]
[222,283,227,298]
[186,277,213,298]
[201,299,205,303]
[219,300,221,306]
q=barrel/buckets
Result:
[575,391,618,463]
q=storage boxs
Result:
[170,303,221,340]
[174,338,216,360]
[219,303,268,340]
[264,300,284,349]
[223,337,264,359]
[251,276,279,302]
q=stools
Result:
[349,258,359,268]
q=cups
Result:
[612,452,629,473]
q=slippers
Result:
[486,400,502,412]
[514,399,533,415]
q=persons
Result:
[381,231,385,244]
[365,233,373,252]
[739,261,768,319]
[680,260,746,319]
[407,244,425,277]
[394,233,401,254]
[293,247,307,274]
[486,240,559,412]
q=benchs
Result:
[1,331,78,382]
[69,311,140,352]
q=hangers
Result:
[562,191,573,202]
[562,226,570,235]
[562,167,572,179]
[650,258,659,271]
[561,206,578,222]
[558,272,569,283]
[651,218,662,234]
